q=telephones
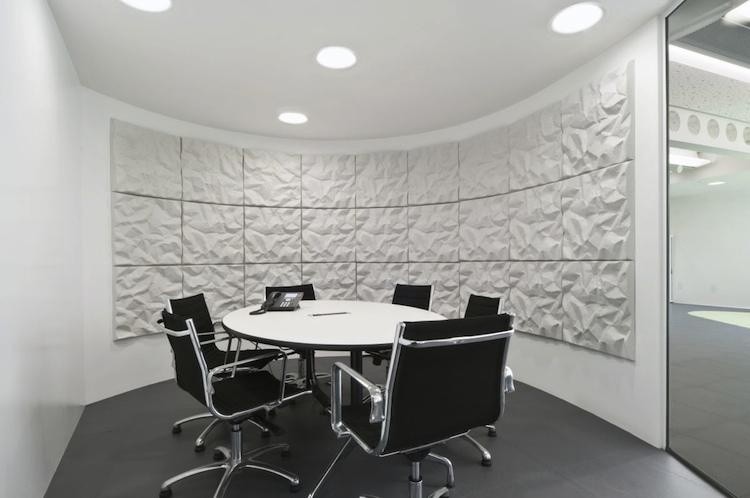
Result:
[260,290,305,311]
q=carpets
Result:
[688,310,750,327]
